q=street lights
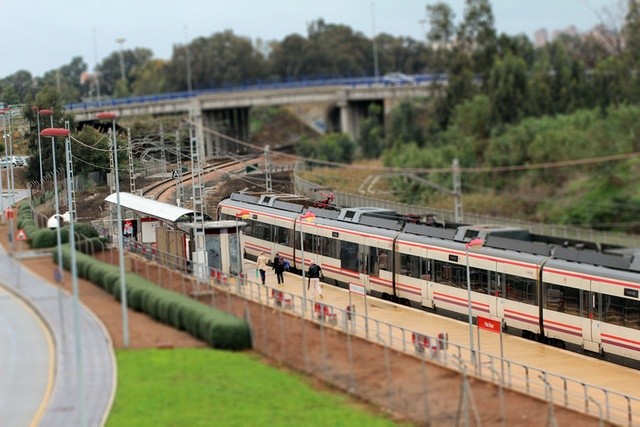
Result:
[0,109,10,190]
[96,111,128,350]
[39,109,64,282]
[301,213,316,311]
[236,211,251,273]
[40,128,79,298]
[31,105,43,189]
[4,107,14,189]
[466,238,485,361]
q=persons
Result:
[308,259,324,299]
[379,248,388,270]
[272,252,284,284]
[256,250,268,285]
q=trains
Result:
[215,191,640,362]
